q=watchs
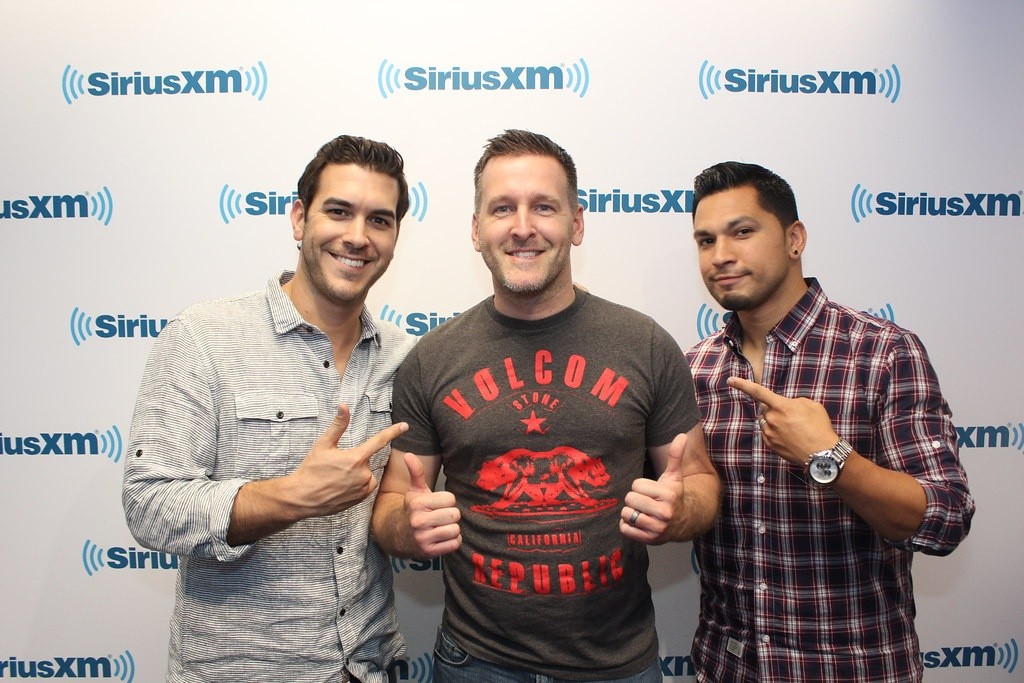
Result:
[804,440,853,485]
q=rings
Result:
[629,509,641,524]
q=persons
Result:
[683,160,975,683]
[369,128,718,683]
[124,134,419,683]
